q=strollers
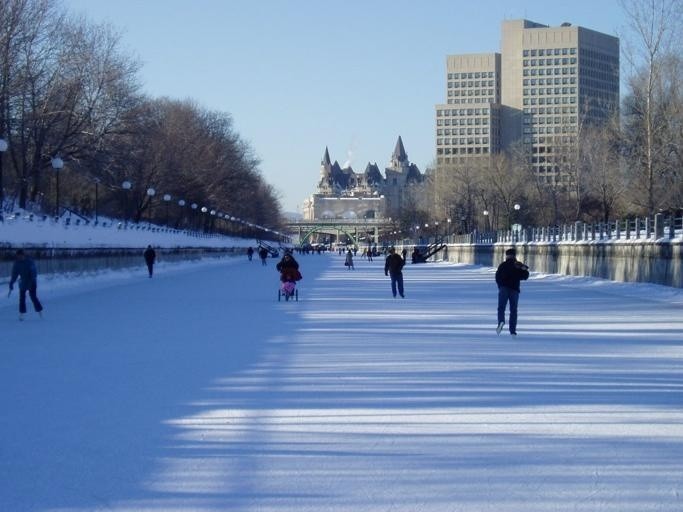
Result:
[277,270,300,306]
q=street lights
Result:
[358,201,522,247]
[51,157,66,225]
[119,178,136,229]
[92,173,102,225]
[141,188,295,244]
[0,137,11,224]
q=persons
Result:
[496,249,529,335]
[401,248,407,261]
[259,248,267,266]
[384,248,405,297]
[10,249,43,321]
[277,253,302,296]
[346,250,354,270]
[247,247,254,261]
[144,245,156,278]
[295,243,325,256]
[338,247,387,261]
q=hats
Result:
[506,249,517,256]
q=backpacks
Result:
[513,262,529,280]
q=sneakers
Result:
[509,331,517,337]
[496,321,505,337]
[20,313,26,321]
[39,310,44,318]
